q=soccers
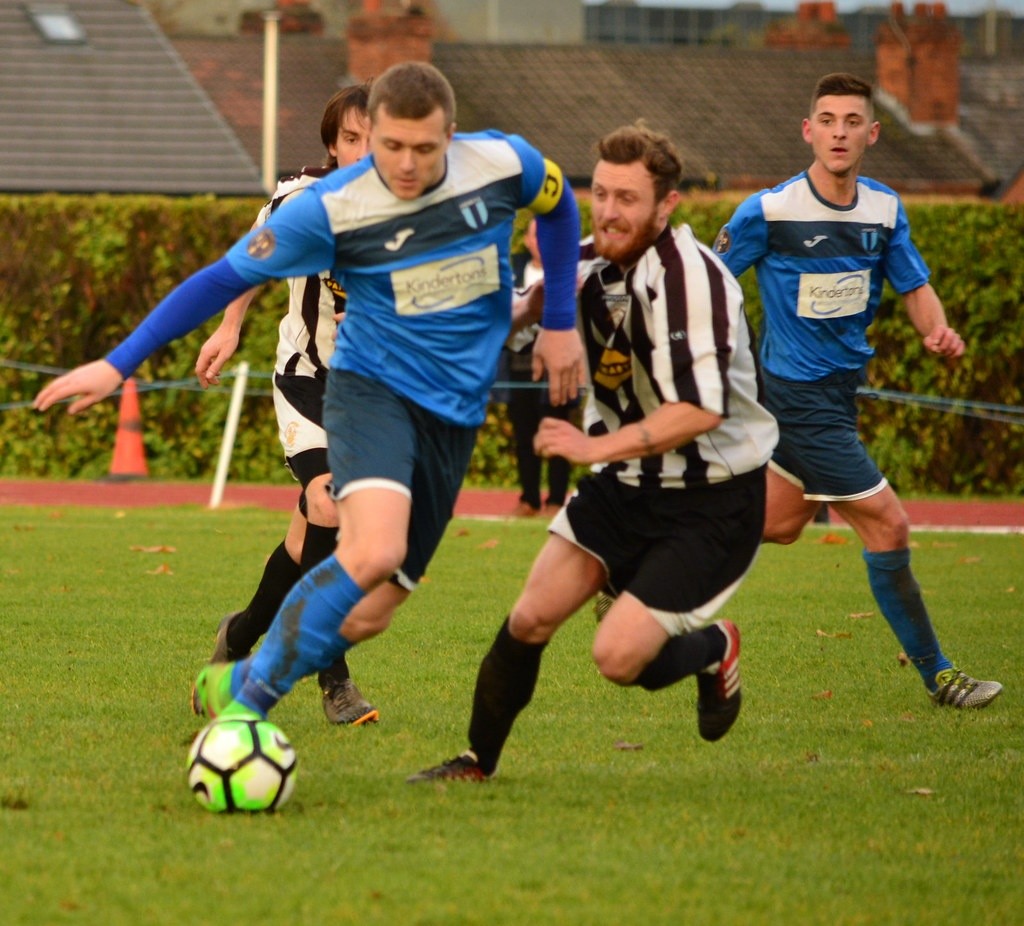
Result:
[185,713,299,813]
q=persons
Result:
[493,216,582,519]
[195,84,380,725]
[34,62,584,718]
[713,72,1004,710]
[407,127,780,782]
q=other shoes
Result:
[514,500,535,518]
[548,501,561,516]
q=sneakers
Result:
[594,591,613,622]
[696,621,742,741]
[193,660,233,721]
[404,747,500,786]
[323,673,378,725]
[218,699,261,722]
[926,667,1002,709]
[192,614,237,720]
